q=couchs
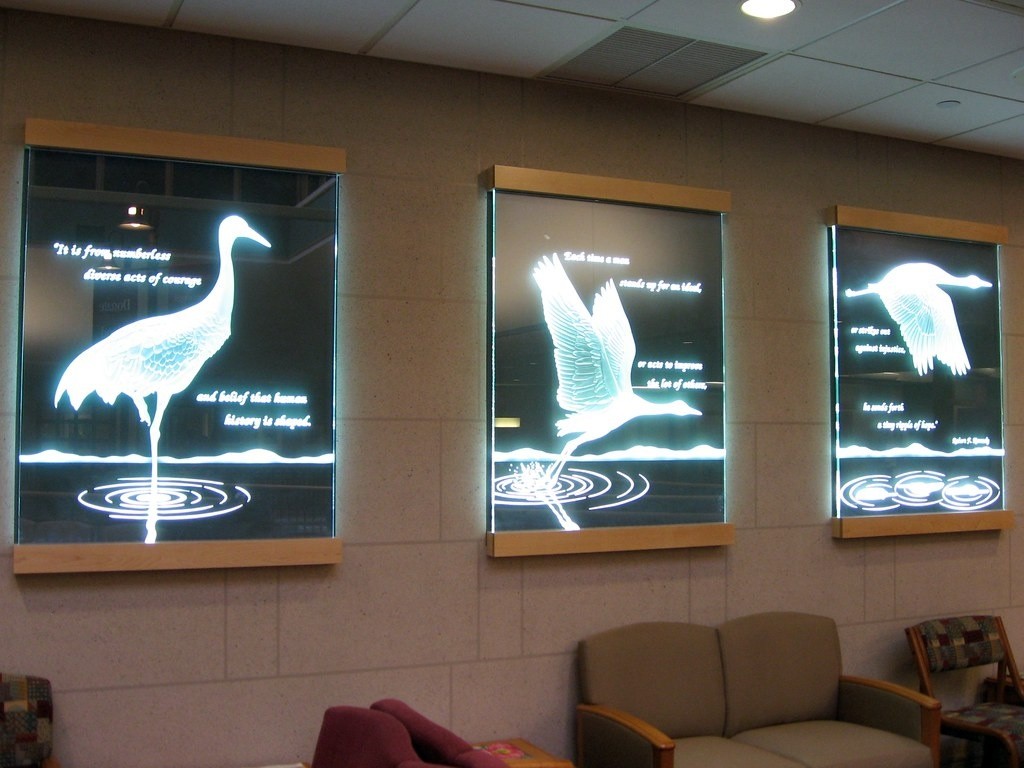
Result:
[577,611,942,768]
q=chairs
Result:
[905,616,1024,768]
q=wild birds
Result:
[844,261,994,377]
[53,215,272,493]
[530,252,703,486]
